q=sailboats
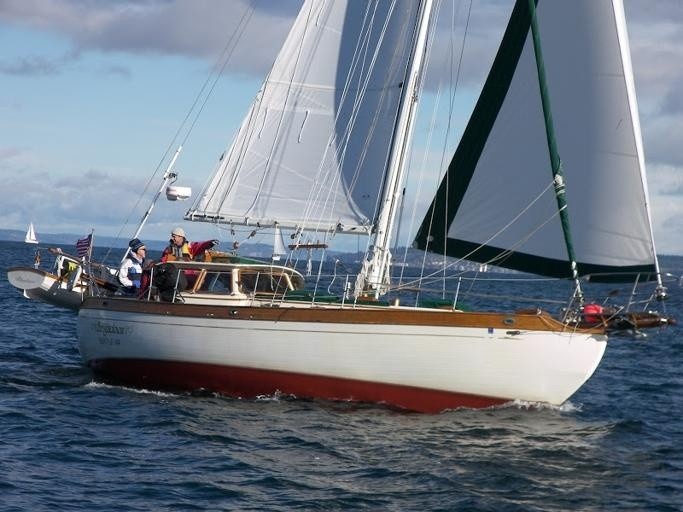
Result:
[269,225,288,264]
[23,222,41,246]
[5,1,681,416]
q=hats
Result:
[170,227,187,238]
[129,238,145,251]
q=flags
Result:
[75,234,91,260]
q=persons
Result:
[157,226,221,287]
[117,236,147,290]
